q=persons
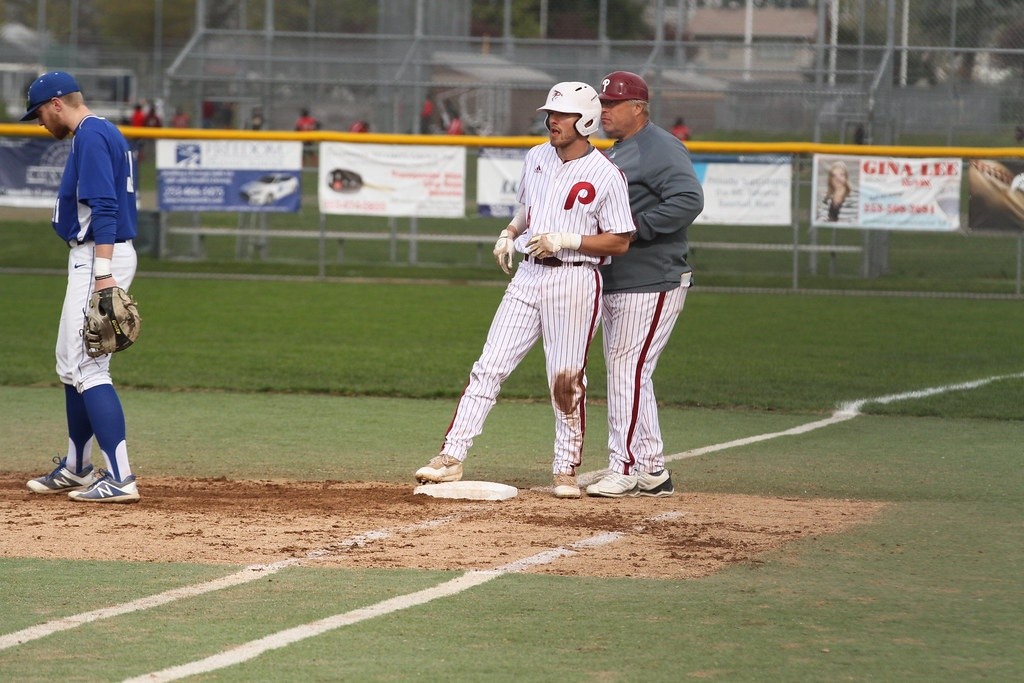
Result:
[173,104,189,128]
[586,71,704,497]
[421,97,475,134]
[350,120,369,133]
[819,162,858,222]
[19,71,141,500]
[672,118,689,139]
[295,107,321,131]
[132,103,162,127]
[416,82,637,497]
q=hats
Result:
[19,71,79,121]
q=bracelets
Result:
[95,257,112,280]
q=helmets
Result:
[597,71,649,101]
[536,81,602,136]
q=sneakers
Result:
[67,468,141,503]
[637,469,674,496]
[553,473,580,499]
[415,453,463,484]
[586,472,640,498]
[26,453,97,494]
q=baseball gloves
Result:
[78,286,144,368]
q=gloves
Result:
[525,232,581,259]
[493,229,516,275]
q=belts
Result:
[524,254,583,267]
[68,237,126,248]
